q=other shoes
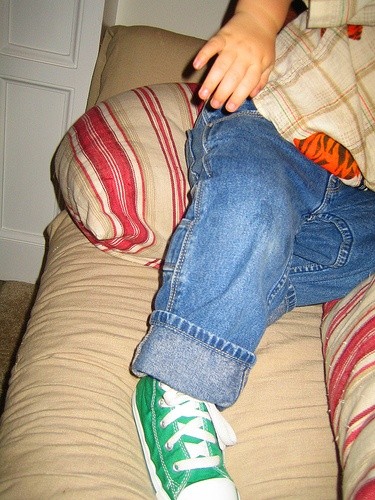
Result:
[132,375,240,496]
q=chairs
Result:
[0,24,375,500]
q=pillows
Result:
[54,82,206,269]
